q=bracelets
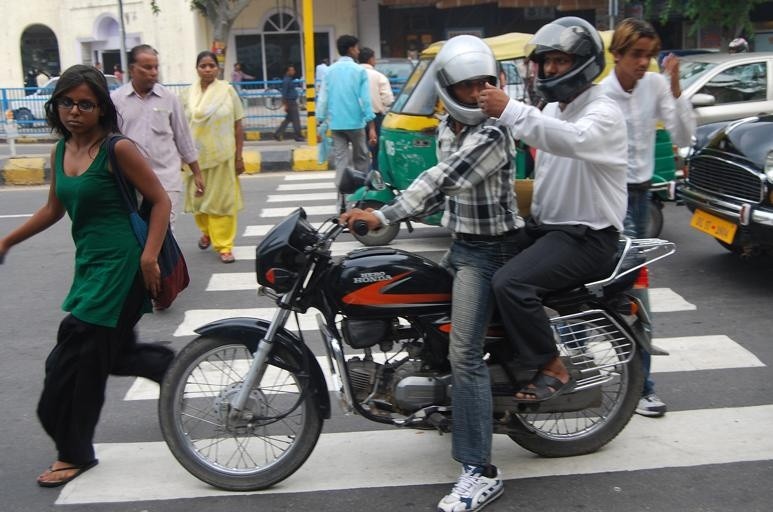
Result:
[236,157,243,161]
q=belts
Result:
[452,233,502,242]
[626,182,650,193]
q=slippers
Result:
[219,251,234,263]
[198,233,210,250]
[37,458,99,488]
[513,366,576,403]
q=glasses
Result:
[58,98,99,114]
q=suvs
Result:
[10,75,123,129]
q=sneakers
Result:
[559,345,593,362]
[436,463,505,512]
[635,393,666,418]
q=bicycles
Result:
[261,78,308,112]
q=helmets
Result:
[431,34,500,128]
[528,15,606,106]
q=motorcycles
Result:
[340,29,678,253]
[151,167,679,492]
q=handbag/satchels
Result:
[107,135,190,311]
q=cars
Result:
[651,43,773,263]
[370,53,532,109]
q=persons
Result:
[0,64,176,487]
[36,67,52,88]
[179,51,247,263]
[551,18,698,419]
[273,33,395,213]
[114,64,124,83]
[109,44,206,311]
[230,63,257,102]
[476,15,629,403]
[728,37,750,53]
[96,63,105,75]
[339,34,527,512]
